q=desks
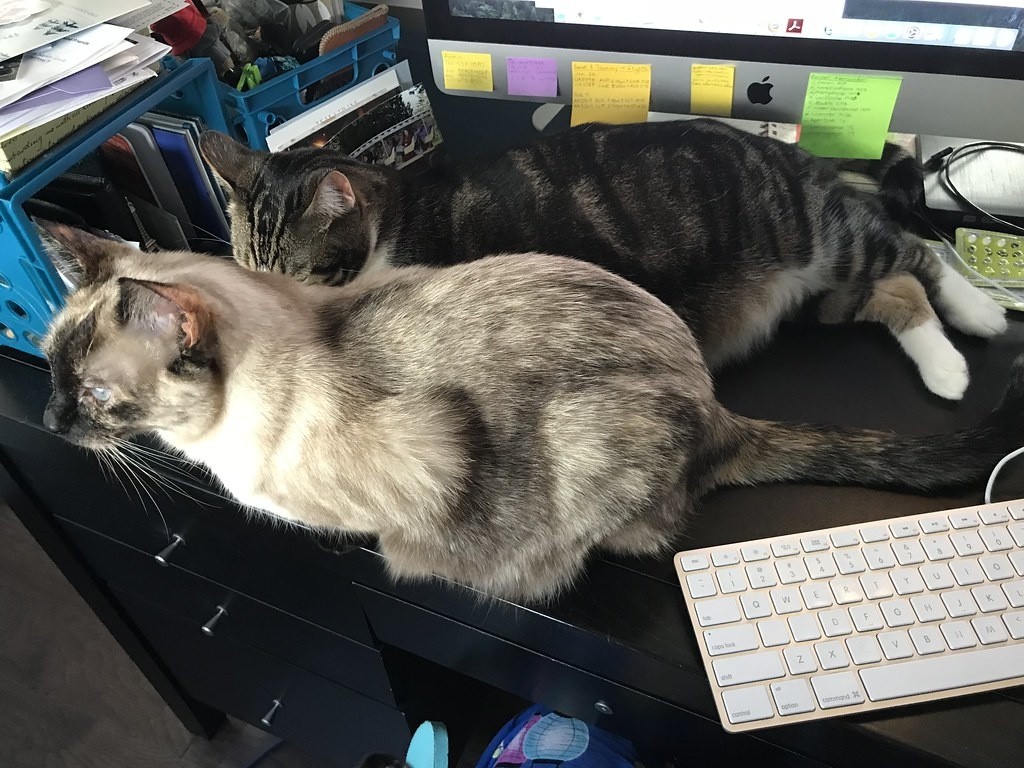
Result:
[0,206,1023,768]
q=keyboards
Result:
[675,496,1024,733]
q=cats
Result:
[197,115,1008,400]
[27,217,1022,608]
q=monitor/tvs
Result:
[421,0,1024,145]
